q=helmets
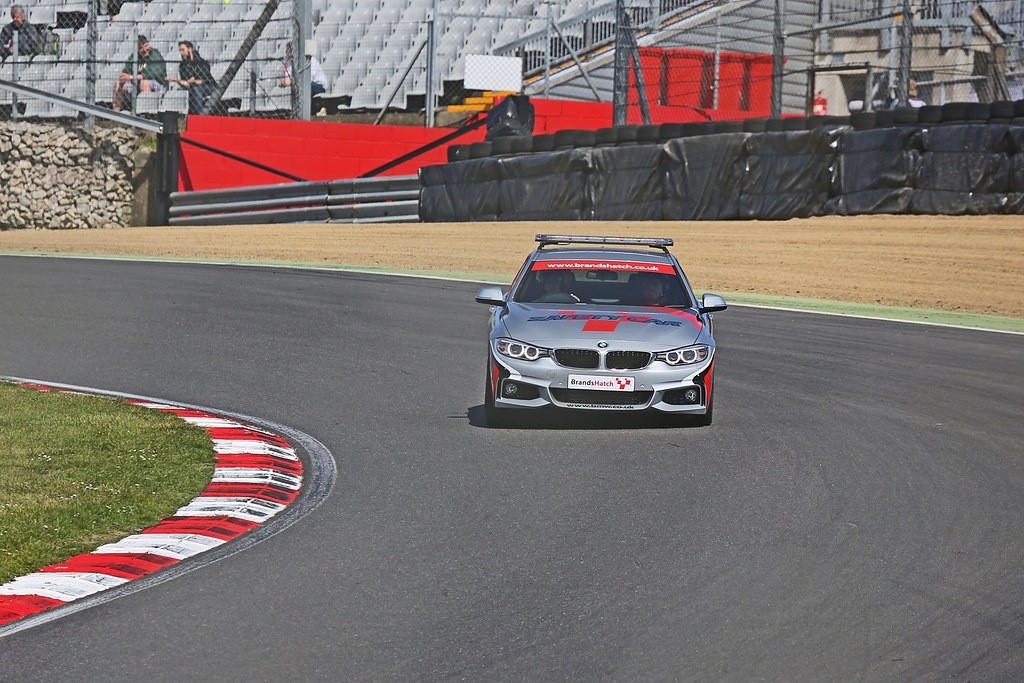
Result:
[536,270,564,286]
[637,270,669,289]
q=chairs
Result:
[619,274,646,306]
[0,0,651,119]
[559,271,576,293]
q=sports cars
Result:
[475,233,730,428]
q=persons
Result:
[0,5,54,62]
[623,275,673,307]
[110,34,222,117]
[277,42,331,113]
[525,271,588,303]
